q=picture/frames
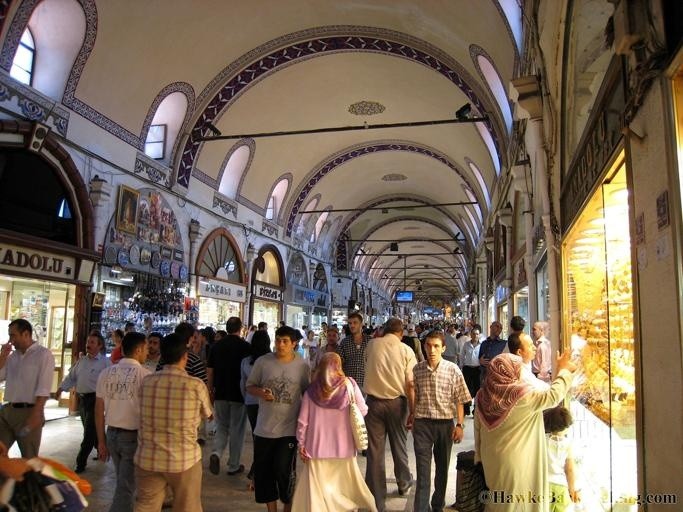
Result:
[115,184,140,235]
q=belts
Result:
[8,401,34,410]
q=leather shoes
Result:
[73,463,85,473]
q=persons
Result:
[1,310,582,512]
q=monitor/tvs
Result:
[395,290,414,304]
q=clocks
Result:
[104,243,188,280]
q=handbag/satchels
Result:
[345,378,369,452]
[543,377,572,433]
[454,450,487,512]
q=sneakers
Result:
[209,455,221,475]
[399,474,414,494]
[227,464,244,475]
